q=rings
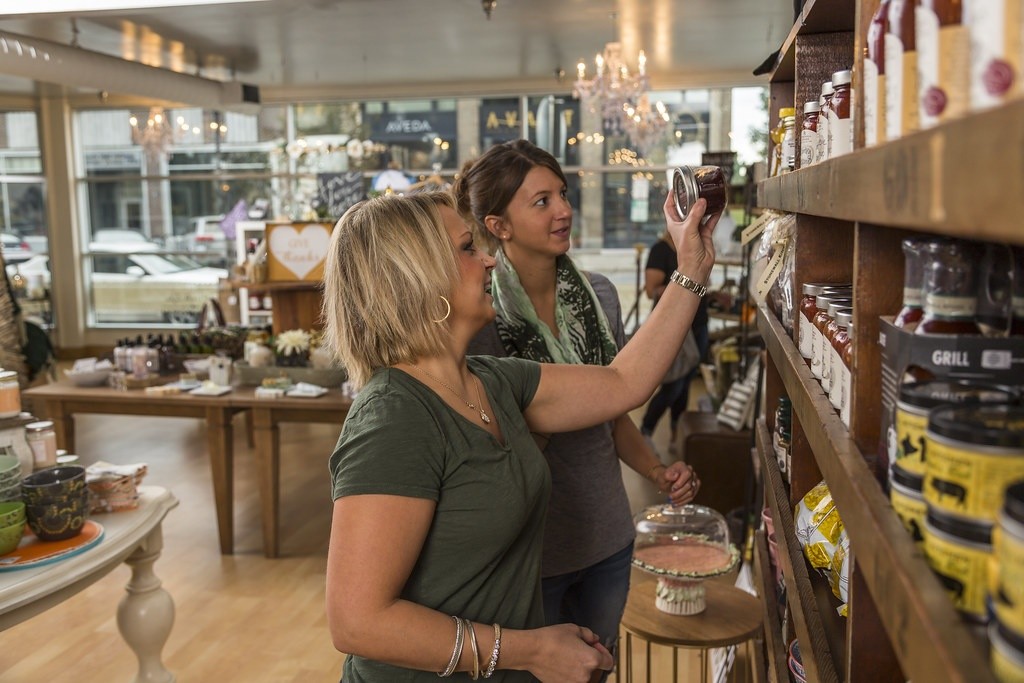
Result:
[692,480,696,489]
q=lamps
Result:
[130,102,196,162]
[621,91,669,148]
[572,12,648,119]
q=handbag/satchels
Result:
[621,285,699,384]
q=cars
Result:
[1,232,33,263]
[4,240,230,323]
[95,228,150,243]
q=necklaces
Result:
[409,362,491,424]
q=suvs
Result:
[173,214,225,257]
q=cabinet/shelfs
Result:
[706,0,1024,683]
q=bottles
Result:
[110,333,212,381]
[24,422,58,469]
[768,1,1024,490]
[0,371,20,420]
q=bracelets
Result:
[437,616,465,678]
[481,623,500,678]
[670,270,707,296]
[647,464,666,494]
[463,618,479,681]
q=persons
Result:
[452,138,703,683]
[640,233,709,458]
[313,185,726,683]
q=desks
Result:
[617,579,763,683]
[20,375,254,556]
[230,385,355,559]
[0,485,181,683]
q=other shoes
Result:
[668,442,677,454]
[644,433,661,457]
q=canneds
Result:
[671,165,728,220]
[0,368,57,472]
[773,395,792,487]
[770,68,851,179]
[916,401,1024,683]
[798,281,854,432]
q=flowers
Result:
[275,327,314,368]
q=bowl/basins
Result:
[62,368,115,386]
[761,507,806,681]
[0,456,87,556]
